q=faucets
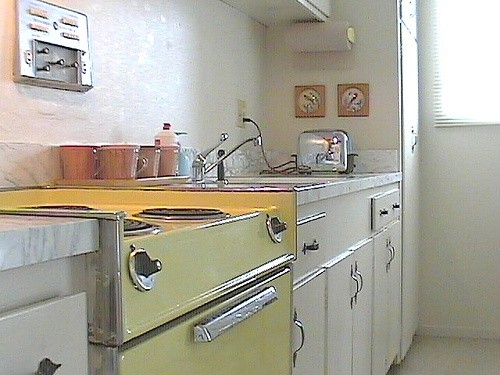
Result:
[190,135,262,183]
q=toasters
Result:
[290,130,358,175]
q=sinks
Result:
[159,181,325,192]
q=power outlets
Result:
[236,98,248,129]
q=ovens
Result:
[88,264,294,375]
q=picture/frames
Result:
[337,83,369,117]
[294,84,325,118]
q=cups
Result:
[159,146,179,177]
[98,144,148,180]
[60,145,105,179]
[137,145,161,178]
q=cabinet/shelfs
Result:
[294,182,404,375]
[221,0,331,24]
[397,0,421,366]
[1,251,100,375]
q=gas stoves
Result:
[1,185,297,346]
